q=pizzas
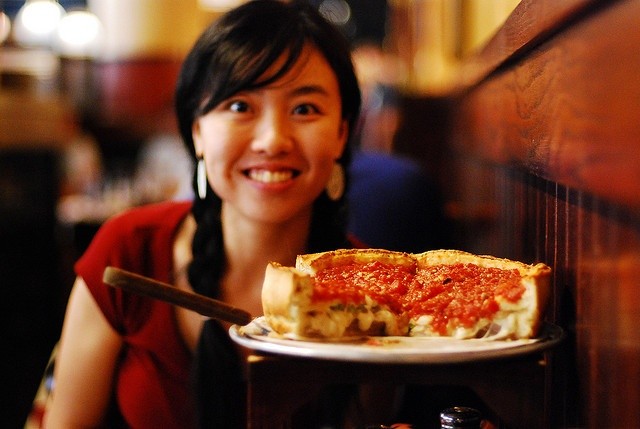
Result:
[263,248,554,344]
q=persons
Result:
[40,0,371,428]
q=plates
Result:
[228,321,564,364]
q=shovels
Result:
[101,266,367,343]
